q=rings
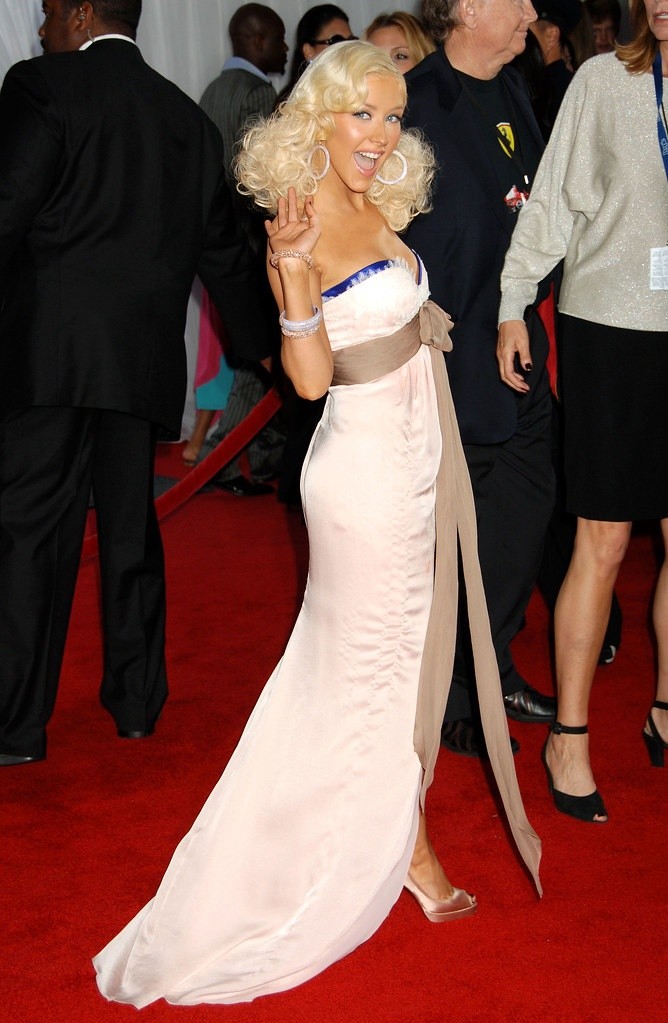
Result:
[300,218,309,223]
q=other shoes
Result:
[0,754,46,766]
[598,644,617,665]
[441,720,520,759]
[503,687,558,722]
[116,711,162,737]
[182,439,202,467]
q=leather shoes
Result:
[252,469,280,482]
[209,476,275,496]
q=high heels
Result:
[402,871,478,922]
[541,720,609,823]
[641,700,668,768]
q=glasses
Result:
[312,34,360,45]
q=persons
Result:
[94,36,541,1009]
[0,0,227,765]
[202,0,668,825]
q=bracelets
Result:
[282,325,320,338]
[269,249,314,269]
[279,305,321,331]
